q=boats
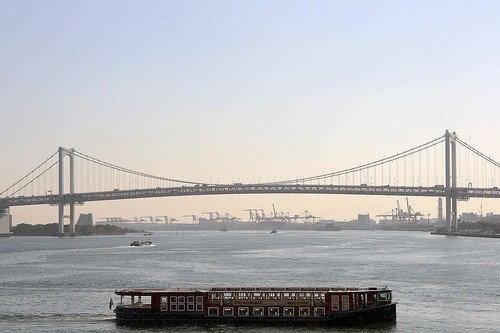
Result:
[271,230,277,233]
[130,241,152,247]
[144,232,151,235]
[113,284,397,329]
